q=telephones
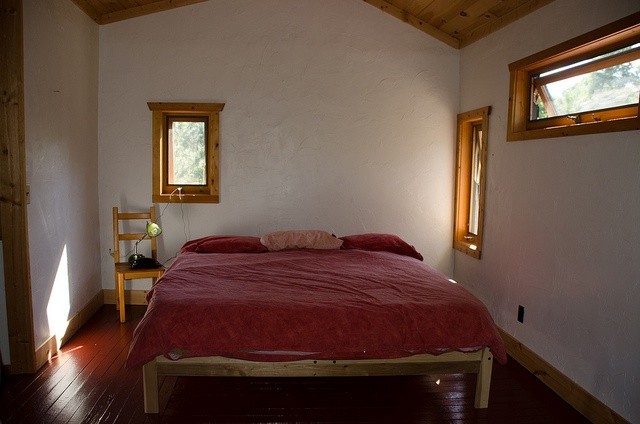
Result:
[131,258,161,270]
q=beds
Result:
[142,235,493,413]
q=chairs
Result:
[113,207,166,323]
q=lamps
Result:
[129,221,162,270]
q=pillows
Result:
[261,231,344,251]
[181,235,268,253]
[339,234,423,261]
